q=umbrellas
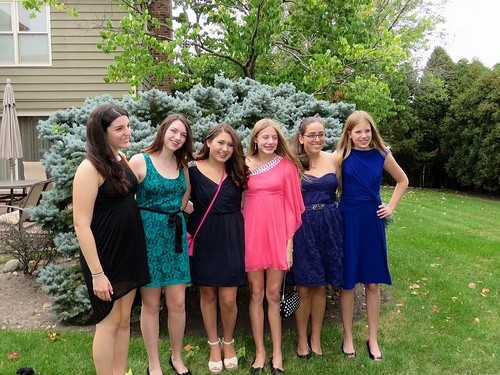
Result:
[0,78,23,183]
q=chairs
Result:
[0,160,54,231]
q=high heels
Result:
[147,366,164,375]
[366,340,383,361]
[297,335,324,361]
[341,340,357,359]
[207,337,239,373]
[169,356,193,375]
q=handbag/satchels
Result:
[187,232,194,256]
[281,270,301,318]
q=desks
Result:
[0,180,41,212]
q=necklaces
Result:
[208,161,223,174]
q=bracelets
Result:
[91,271,104,276]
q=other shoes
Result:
[249,356,285,375]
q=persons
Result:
[286,116,342,359]
[72,102,152,375]
[181,123,250,373]
[127,113,192,375]
[333,110,409,363]
[242,117,306,375]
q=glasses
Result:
[302,133,326,139]
